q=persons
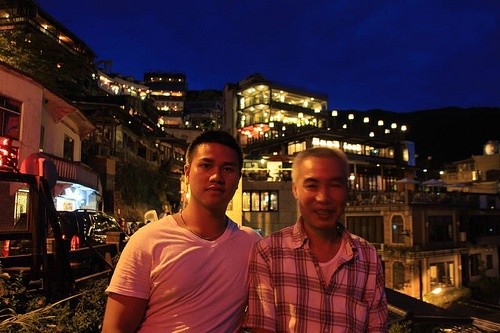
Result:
[243,145,388,333]
[100,129,264,333]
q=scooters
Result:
[128,216,146,235]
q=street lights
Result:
[419,258,442,302]
[178,191,189,208]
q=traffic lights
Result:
[122,218,125,230]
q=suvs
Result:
[0,209,129,280]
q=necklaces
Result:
[180,208,228,240]
